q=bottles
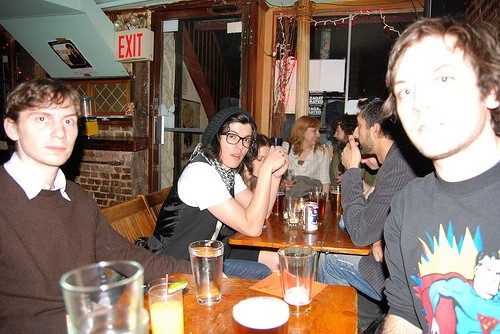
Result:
[81,96,93,117]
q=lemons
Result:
[168,280,188,293]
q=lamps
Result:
[113,14,154,61]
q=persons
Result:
[228,135,282,273]
[65,43,85,64]
[329,112,383,198]
[144,108,290,281]
[382,8,500,334]
[0,76,192,334]
[317,97,417,301]
[279,115,331,202]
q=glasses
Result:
[217,132,255,148]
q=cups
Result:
[147,278,186,334]
[272,192,286,217]
[231,296,291,334]
[286,196,308,232]
[59,259,144,334]
[307,191,326,225]
[188,239,223,306]
[278,244,317,319]
[329,184,343,216]
[284,169,295,184]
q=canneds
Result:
[304,201,318,231]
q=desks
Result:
[142,270,360,334]
[228,199,374,256]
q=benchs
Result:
[100,186,173,242]
